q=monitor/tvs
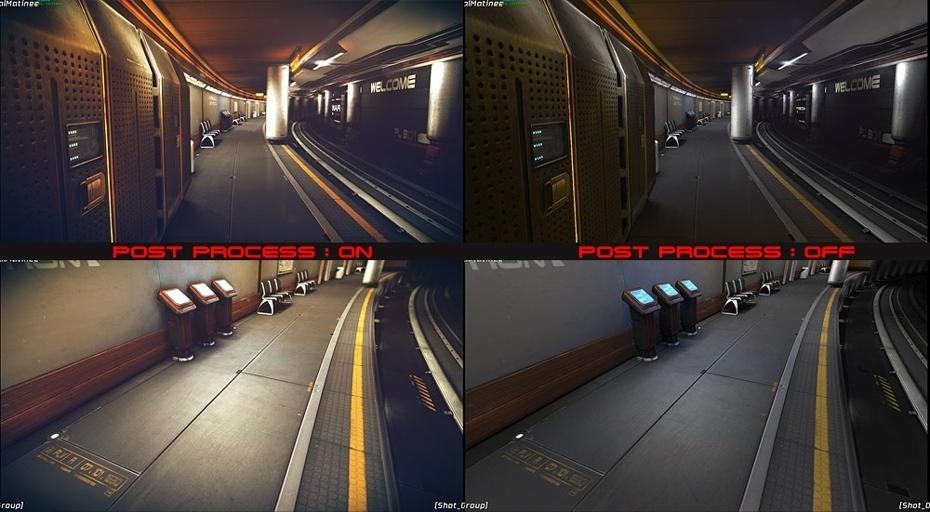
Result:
[680,280,698,292]
[629,288,655,306]
[215,278,234,293]
[163,288,192,306]
[658,283,680,298]
[192,283,214,298]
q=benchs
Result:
[703,113,713,121]
[696,115,706,125]
[759,269,781,297]
[662,119,685,149]
[294,269,317,296]
[821,262,831,272]
[232,113,240,128]
[257,277,292,316]
[197,121,221,150]
[720,277,756,316]
[356,265,367,273]
[240,113,247,122]
